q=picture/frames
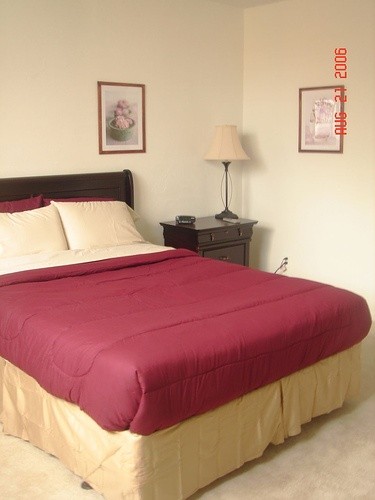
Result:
[299,85,344,153]
[97,81,146,154]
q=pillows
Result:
[0,194,150,257]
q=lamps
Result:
[205,125,248,219]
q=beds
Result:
[0,170,371,500]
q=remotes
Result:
[223,217,240,224]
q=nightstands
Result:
[159,216,258,267]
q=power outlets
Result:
[280,255,290,273]
